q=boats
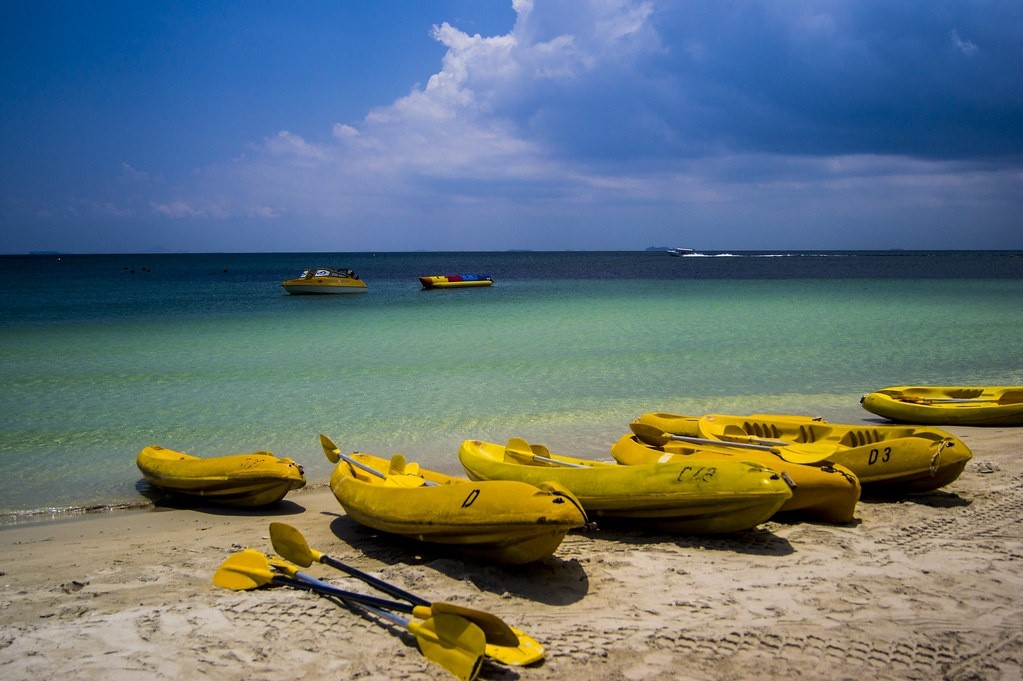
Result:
[136,446,306,511]
[860,384,1022,428]
[280,268,368,296]
[329,450,599,567]
[609,412,972,526]
[457,437,795,535]
[667,247,694,257]
[418,272,494,289]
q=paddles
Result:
[892,391,1023,405]
[502,437,592,468]
[213,522,545,681]
[320,433,440,487]
[629,423,838,464]
